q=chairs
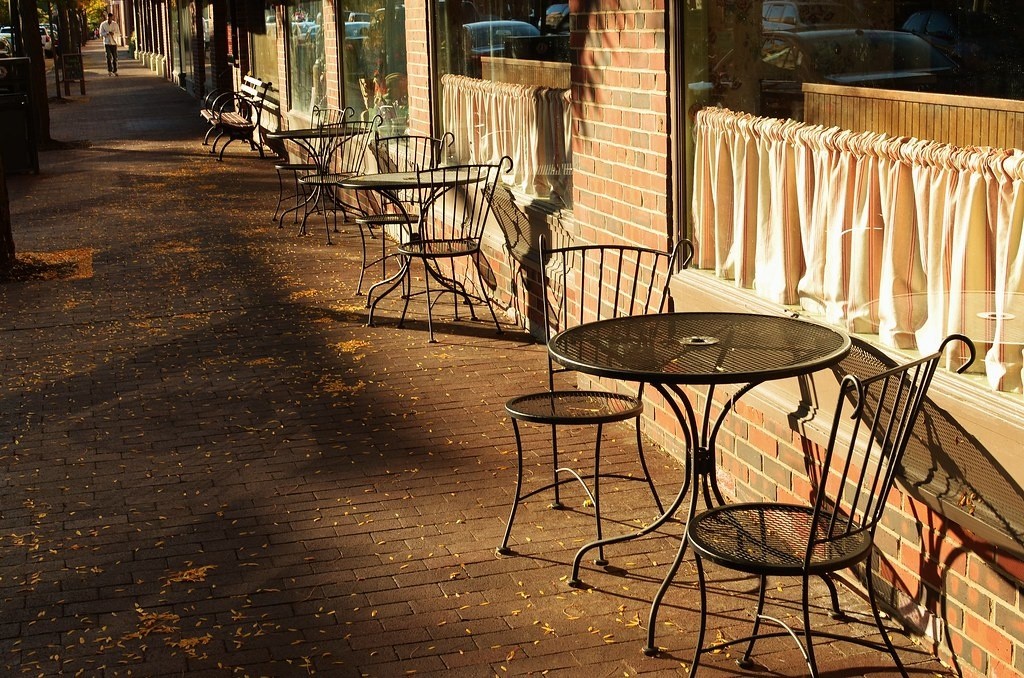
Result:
[495,231,693,567]
[356,131,455,298]
[273,105,355,222]
[685,333,978,677]
[279,114,384,245]
[364,156,514,343]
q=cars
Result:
[756,1,1023,129]
[448,1,571,91]
[196,4,407,91]
[0,22,58,67]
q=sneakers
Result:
[114,72,118,76]
[107,71,112,76]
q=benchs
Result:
[200,75,273,161]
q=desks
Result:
[544,312,850,654]
[337,169,488,326]
[266,126,377,227]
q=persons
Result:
[99,13,120,77]
